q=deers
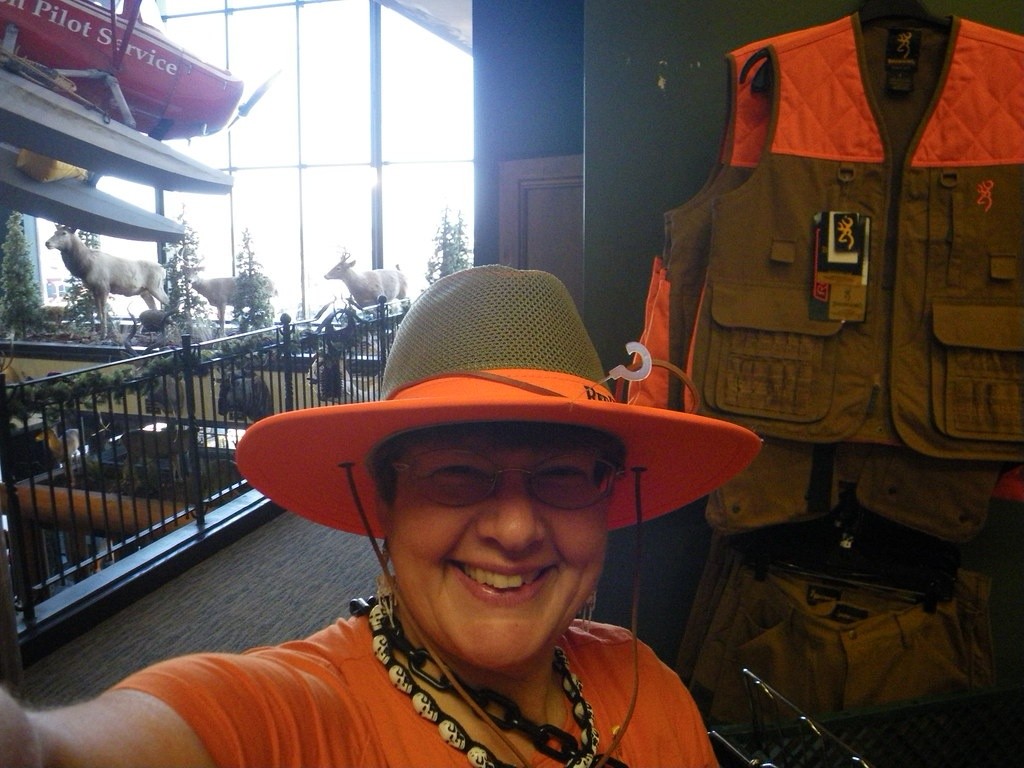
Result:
[43,215,177,340]
[34,426,86,470]
[325,256,407,311]
[173,254,273,340]
[119,421,200,484]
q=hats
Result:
[236,265,762,539]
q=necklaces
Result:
[369,602,601,767]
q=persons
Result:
[0,264,762,768]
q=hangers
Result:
[739,0,952,92]
[754,510,952,615]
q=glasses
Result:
[387,447,625,510]
